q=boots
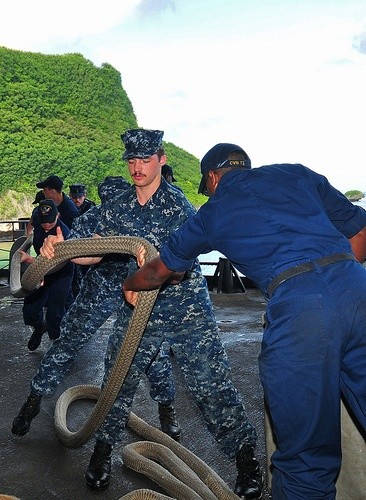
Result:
[234,449,262,500]
[85,442,111,489]
[13,392,42,435]
[159,402,181,440]
[28,319,47,350]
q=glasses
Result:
[202,172,216,196]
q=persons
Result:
[161,164,184,194]
[40,129,264,500]
[17,199,74,351]
[11,176,183,439]
[69,184,96,215]
[32,190,46,204]
[122,142,366,500]
[36,176,84,313]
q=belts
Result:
[267,252,359,298]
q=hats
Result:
[161,164,177,182]
[198,143,251,194]
[37,199,57,223]
[36,175,62,189]
[120,128,164,158]
[98,181,131,202]
[32,190,45,204]
[70,185,85,197]
